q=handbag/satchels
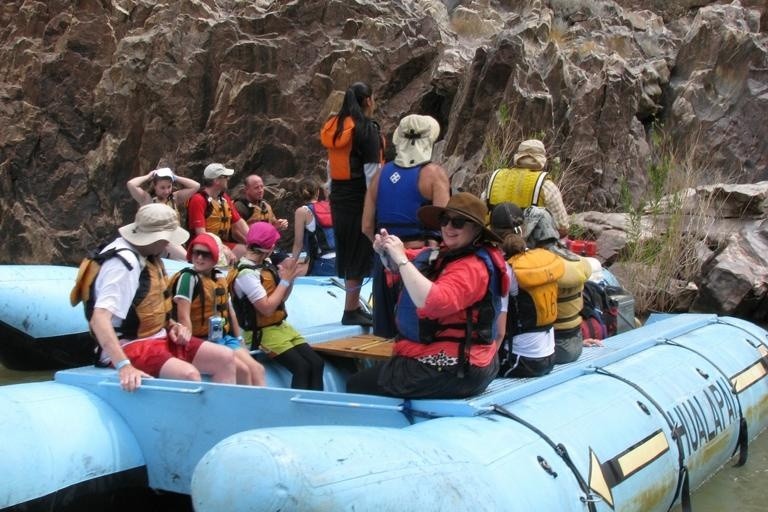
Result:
[353,120,381,162]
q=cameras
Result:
[208,315,224,342]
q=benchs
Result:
[107,309,372,371]
[407,310,722,420]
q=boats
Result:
[0,249,371,371]
[0,296,768,512]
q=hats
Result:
[204,163,235,179]
[154,168,176,182]
[393,114,440,168]
[118,203,222,262]
[514,140,546,170]
[417,192,524,243]
[247,222,281,249]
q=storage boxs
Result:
[567,237,598,261]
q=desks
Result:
[308,332,395,361]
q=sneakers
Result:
[342,307,373,326]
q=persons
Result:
[70,80,604,400]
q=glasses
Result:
[192,249,211,259]
[440,213,470,228]
